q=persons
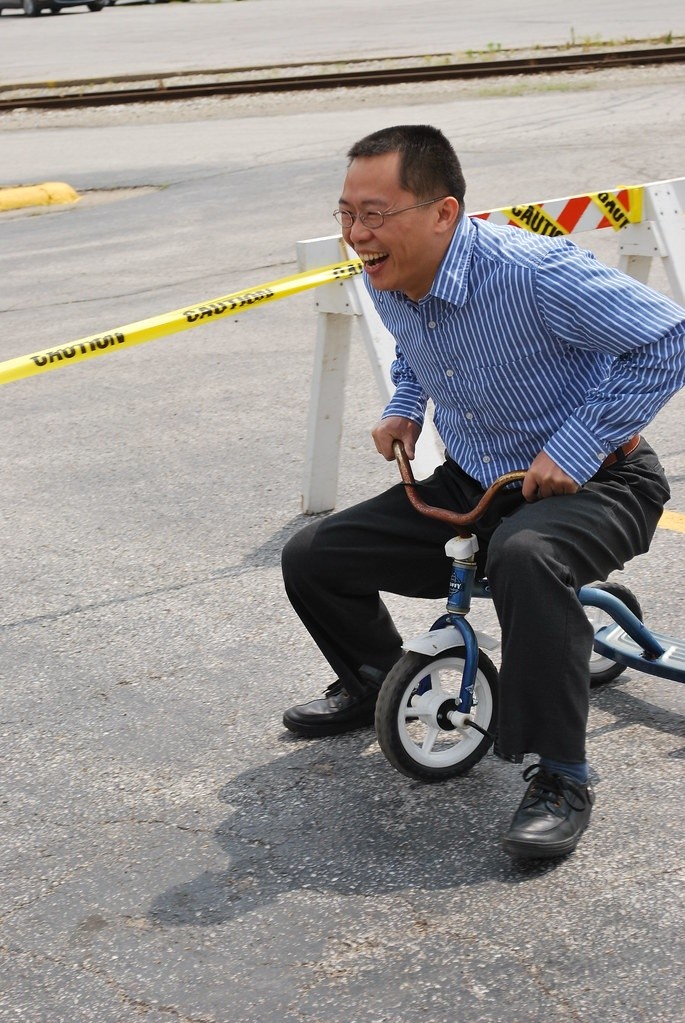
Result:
[281,125,685,859]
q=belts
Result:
[597,433,641,469]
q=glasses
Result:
[332,195,447,229]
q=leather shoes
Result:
[501,763,596,858]
[282,679,380,738]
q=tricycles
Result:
[375,439,685,784]
